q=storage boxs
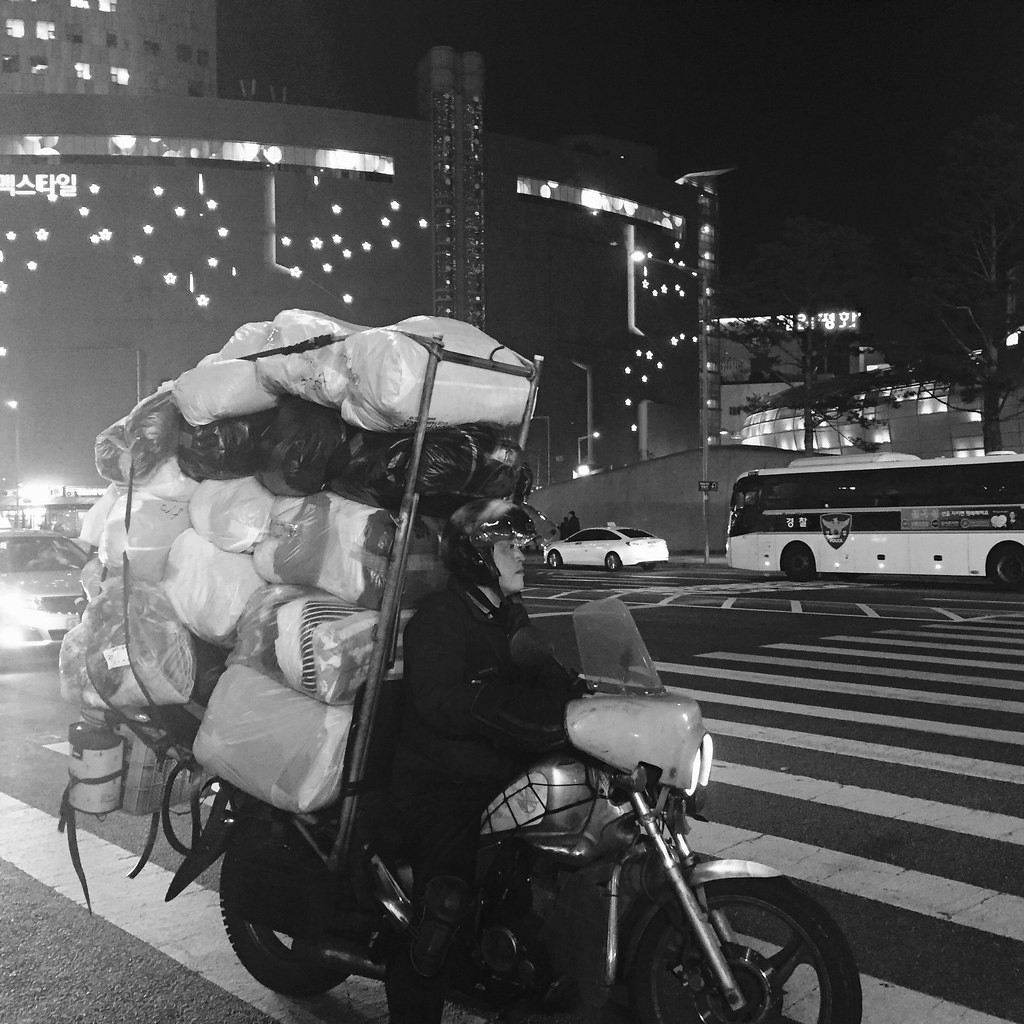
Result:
[79,708,213,817]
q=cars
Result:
[543,521,670,572]
[0,527,92,649]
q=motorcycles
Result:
[139,596,867,1024]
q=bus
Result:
[724,447,1024,591]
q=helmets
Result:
[440,497,533,586]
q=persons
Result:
[25,542,72,572]
[547,510,581,544]
[384,498,609,1024]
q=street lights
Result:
[533,415,551,486]
[576,431,601,465]
[569,357,595,474]
